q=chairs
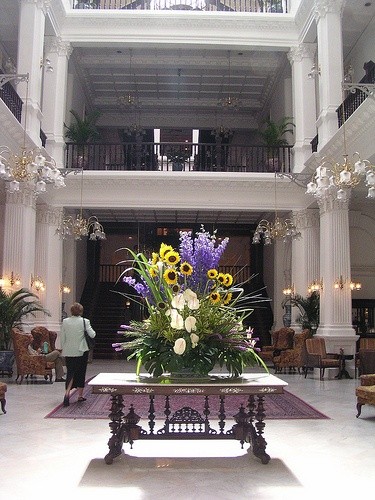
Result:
[258,328,375,418]
[11,326,62,385]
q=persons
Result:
[28,332,67,382]
[55,302,96,406]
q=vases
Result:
[62,302,67,321]
[282,305,291,327]
[164,361,212,379]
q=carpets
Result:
[43,374,334,420]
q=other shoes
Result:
[64,395,69,407]
[78,397,86,401]
[55,377,66,382]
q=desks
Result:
[88,372,288,464]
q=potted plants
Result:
[64,109,104,169]
[255,116,297,173]
[0,288,52,371]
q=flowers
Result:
[110,224,270,376]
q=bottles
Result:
[43,340,49,354]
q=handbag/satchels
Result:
[83,317,96,350]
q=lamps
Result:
[304,82,375,201]
[0,271,22,298]
[40,57,53,73]
[210,49,241,138]
[116,48,147,137]
[252,170,313,245]
[335,274,346,290]
[37,166,107,241]
[0,73,67,196]
[306,62,320,79]
